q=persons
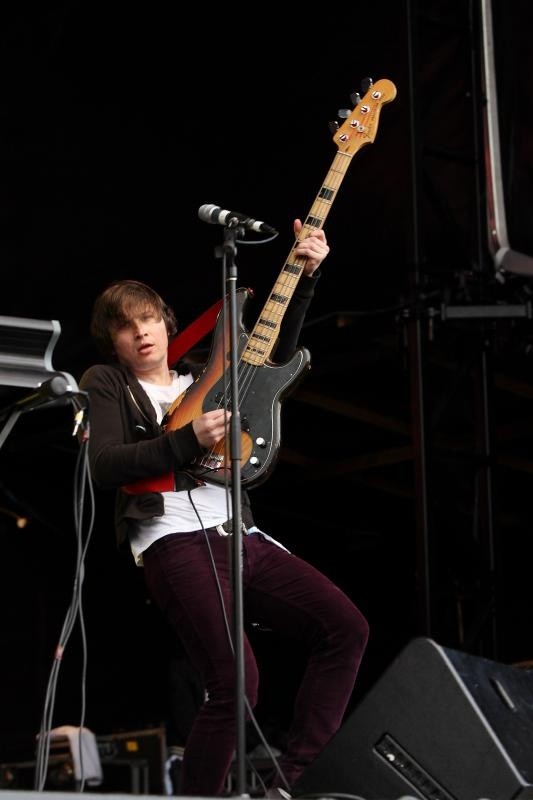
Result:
[77,218,369,799]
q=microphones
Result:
[198,203,279,236]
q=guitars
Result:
[165,78,399,486]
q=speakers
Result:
[281,637,533,799]
